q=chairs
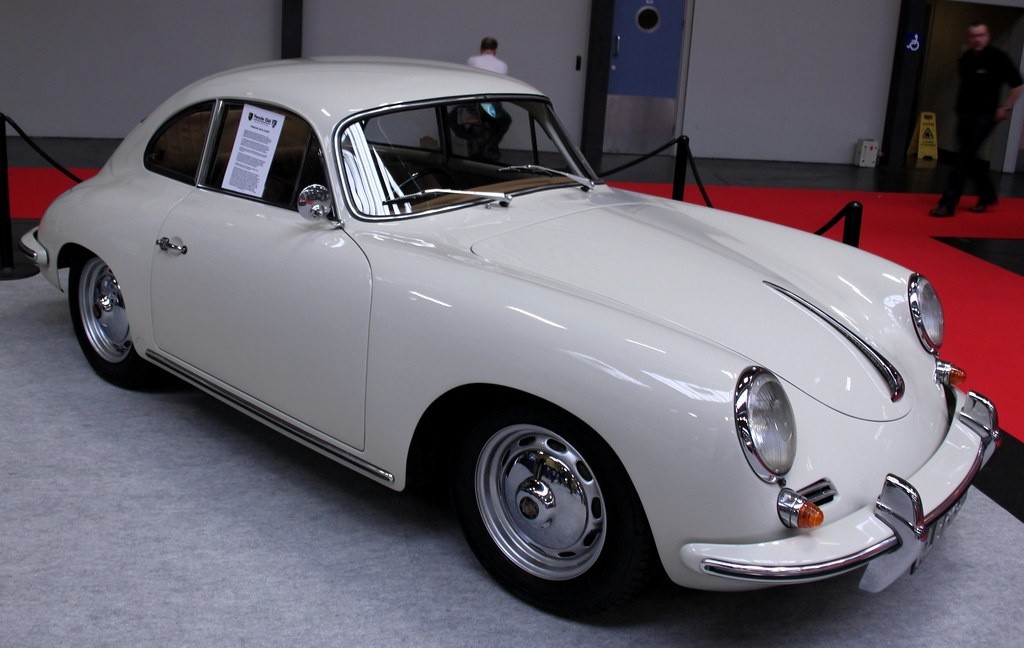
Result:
[384,153,433,206]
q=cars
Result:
[14,55,1004,621]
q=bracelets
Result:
[1004,105,1012,112]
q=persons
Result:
[448,37,512,161]
[929,20,1023,218]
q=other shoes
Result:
[969,196,998,212]
[929,208,954,217]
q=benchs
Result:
[214,144,329,213]
